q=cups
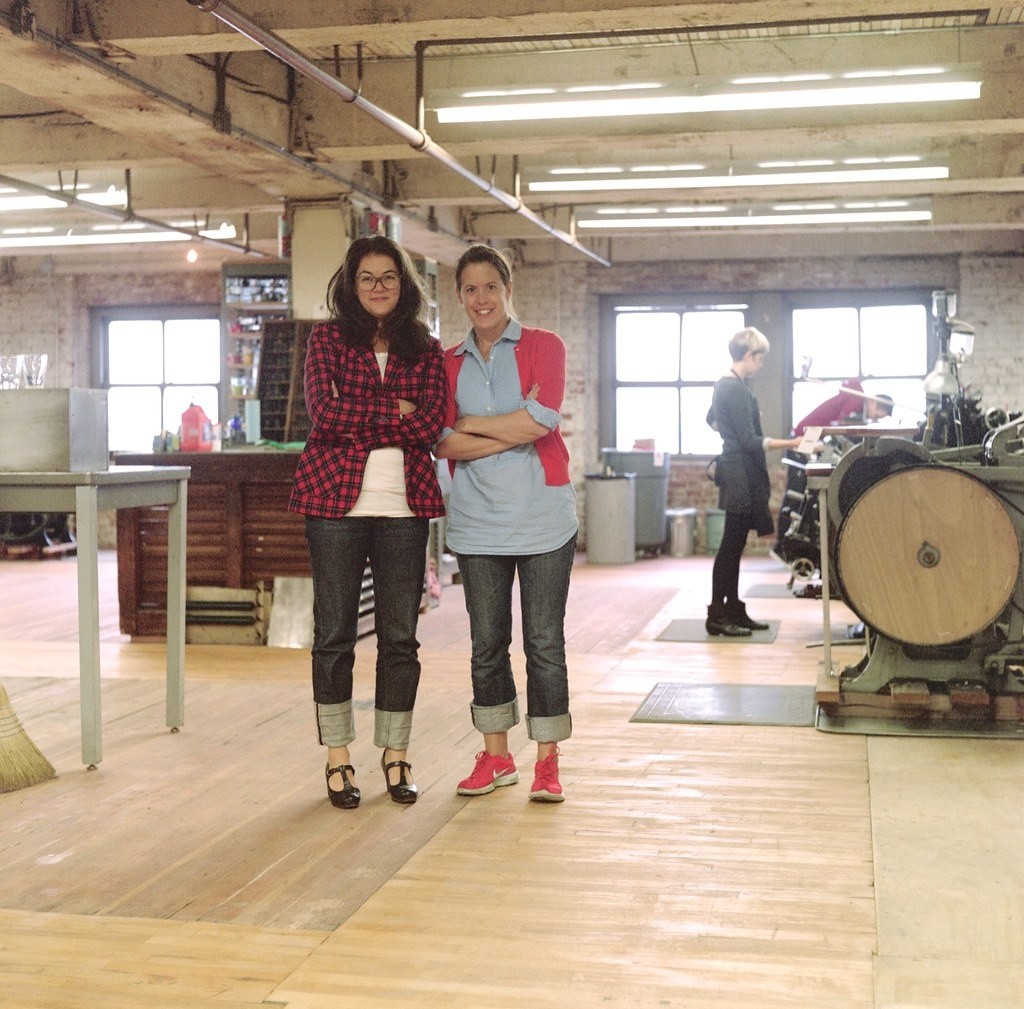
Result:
[23,353,48,388]
[0,355,23,389]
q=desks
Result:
[0,466,193,769]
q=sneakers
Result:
[529,744,565,802]
[457,749,520,796]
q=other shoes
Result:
[770,541,796,565]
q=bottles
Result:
[227,413,242,437]
[182,403,212,451]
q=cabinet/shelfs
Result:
[218,256,292,434]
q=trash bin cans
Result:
[584,473,635,566]
[664,507,697,560]
[600,447,670,558]
[706,510,727,555]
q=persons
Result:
[287,236,447,808]
[706,327,823,636]
[770,381,893,563]
[430,244,580,801]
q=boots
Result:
[725,601,769,631]
[706,603,752,637]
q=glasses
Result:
[354,274,403,291]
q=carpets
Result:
[747,583,804,599]
[655,618,781,644]
[628,678,815,729]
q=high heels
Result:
[325,761,360,808]
[380,749,418,803]
